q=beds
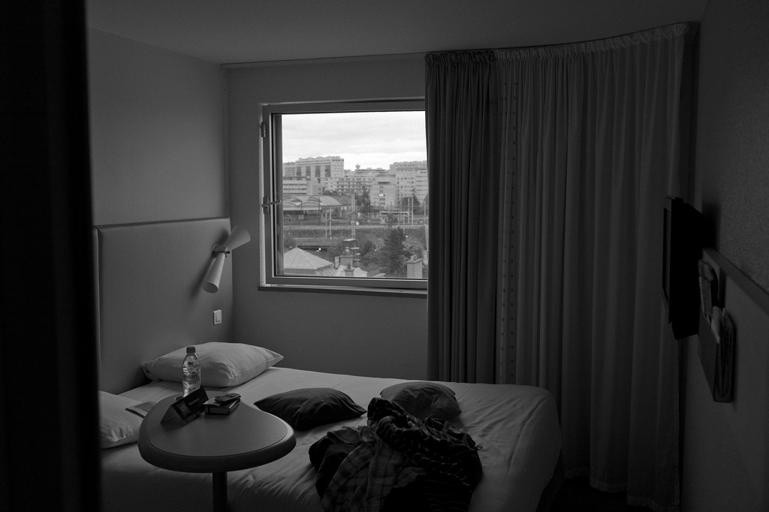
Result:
[92,211,562,509]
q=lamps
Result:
[201,223,254,294]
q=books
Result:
[697,261,733,401]
[127,401,158,418]
[203,396,239,414]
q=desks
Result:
[138,390,297,509]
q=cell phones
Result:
[215,392,241,403]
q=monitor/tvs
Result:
[662,197,710,340]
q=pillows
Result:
[142,341,285,387]
[378,381,462,420]
[253,386,368,431]
[98,388,155,451]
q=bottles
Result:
[181,345,201,398]
